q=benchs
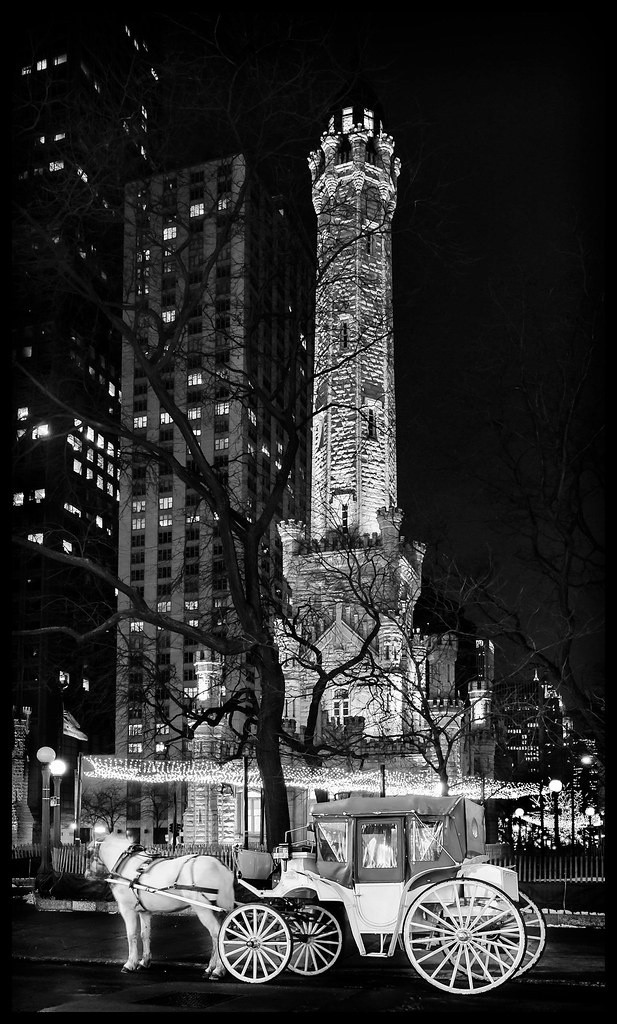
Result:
[272,822,317,859]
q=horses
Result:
[84,832,235,981]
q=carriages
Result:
[85,794,550,996]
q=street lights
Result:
[49,758,69,849]
[586,807,595,860]
[239,741,253,851]
[37,746,57,884]
[548,779,563,852]
[514,808,524,854]
